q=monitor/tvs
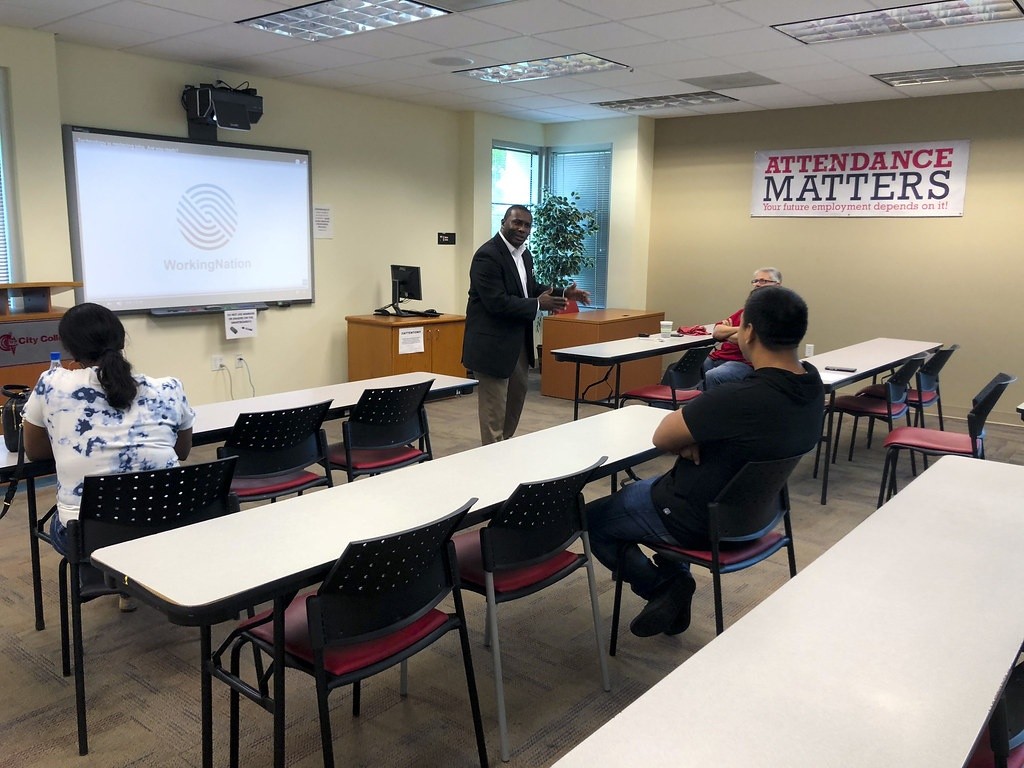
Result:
[391,265,422,316]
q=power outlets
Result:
[805,343,814,357]
[235,353,244,368]
[212,355,226,370]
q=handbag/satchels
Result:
[1,384,31,452]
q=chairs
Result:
[56,344,1016,768]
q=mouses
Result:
[426,309,437,313]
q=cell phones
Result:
[825,366,858,372]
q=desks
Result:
[797,337,943,504]
[553,454,1024,768]
[541,309,666,404]
[550,323,729,421]
[345,312,467,383]
[91,402,676,768]
[0,372,479,630]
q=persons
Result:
[703,268,783,394]
[460,204,591,446]
[23,302,195,612]
[579,283,825,639]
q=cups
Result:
[659,320,673,338]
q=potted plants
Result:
[523,185,601,376]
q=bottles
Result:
[49,353,62,369]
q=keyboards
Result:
[401,309,439,317]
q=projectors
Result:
[187,88,263,131]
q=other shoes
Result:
[629,569,696,637]
[658,574,695,635]
[118,596,142,611]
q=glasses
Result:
[751,278,779,285]
